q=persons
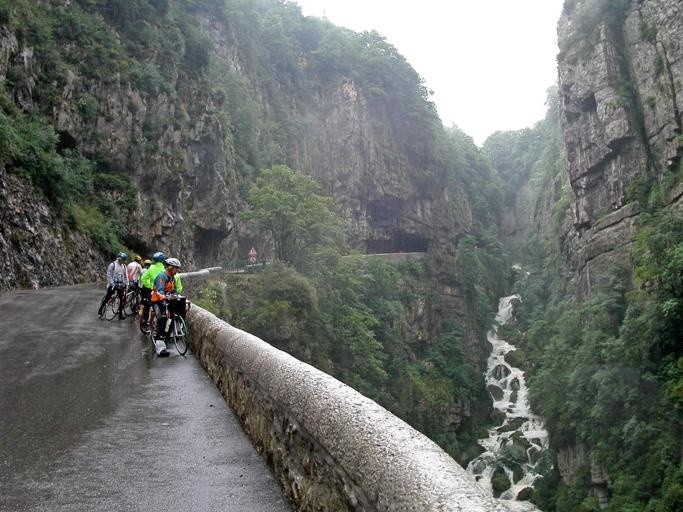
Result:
[97,251,184,355]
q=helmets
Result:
[143,260,151,265]
[116,252,127,261]
[152,252,168,262]
[135,256,141,261]
[164,258,181,269]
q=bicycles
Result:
[94,279,190,356]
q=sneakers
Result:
[141,326,150,334]
[97,314,102,318]
[155,339,166,351]
[118,315,126,320]
